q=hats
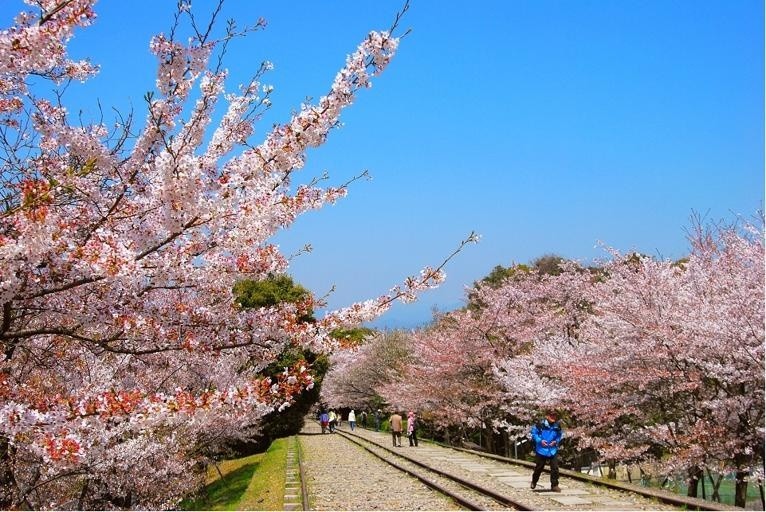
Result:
[546,409,560,416]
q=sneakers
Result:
[531,482,536,489]
[552,486,560,492]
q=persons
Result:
[319,408,329,433]
[327,407,337,433]
[407,410,418,446]
[360,409,367,429]
[348,409,357,431]
[388,408,403,446]
[375,408,383,431]
[528,408,561,492]
[336,409,343,425]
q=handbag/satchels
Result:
[322,420,328,427]
